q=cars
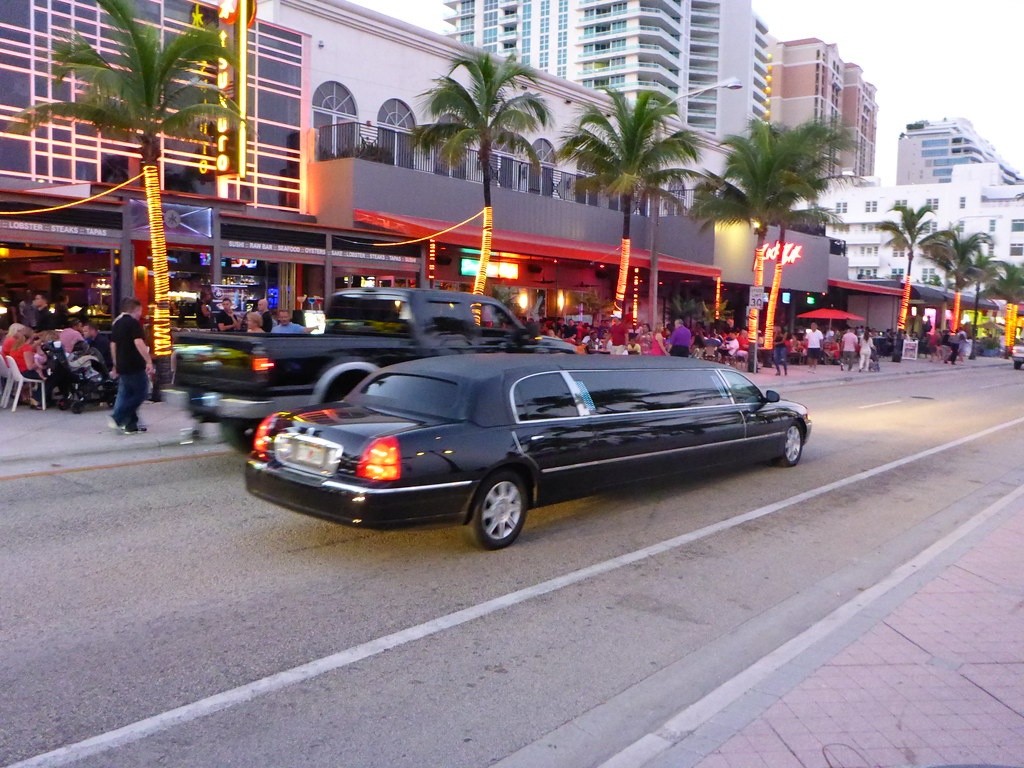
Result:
[243,352,814,550]
[1012,341,1024,370]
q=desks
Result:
[589,348,637,356]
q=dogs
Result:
[868,358,879,372]
[933,344,964,365]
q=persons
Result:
[258,298,273,332]
[0,322,55,410]
[757,322,841,375]
[521,317,629,355]
[270,310,320,332]
[840,325,878,371]
[53,292,71,329]
[196,288,215,328]
[108,297,156,434]
[629,318,761,370]
[216,298,241,332]
[59,317,113,409]
[170,299,186,315]
[33,291,58,331]
[883,315,971,365]
[246,312,266,333]
[19,289,36,327]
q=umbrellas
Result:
[796,304,867,331]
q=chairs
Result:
[0,355,46,412]
[689,334,808,374]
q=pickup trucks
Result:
[170,287,579,457]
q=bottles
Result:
[221,277,236,285]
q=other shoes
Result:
[108,414,122,429]
[125,426,146,434]
[775,371,781,376]
[31,404,42,410]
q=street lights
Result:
[648,78,743,334]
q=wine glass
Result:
[298,296,323,310]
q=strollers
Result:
[56,340,119,414]
[868,349,880,371]
